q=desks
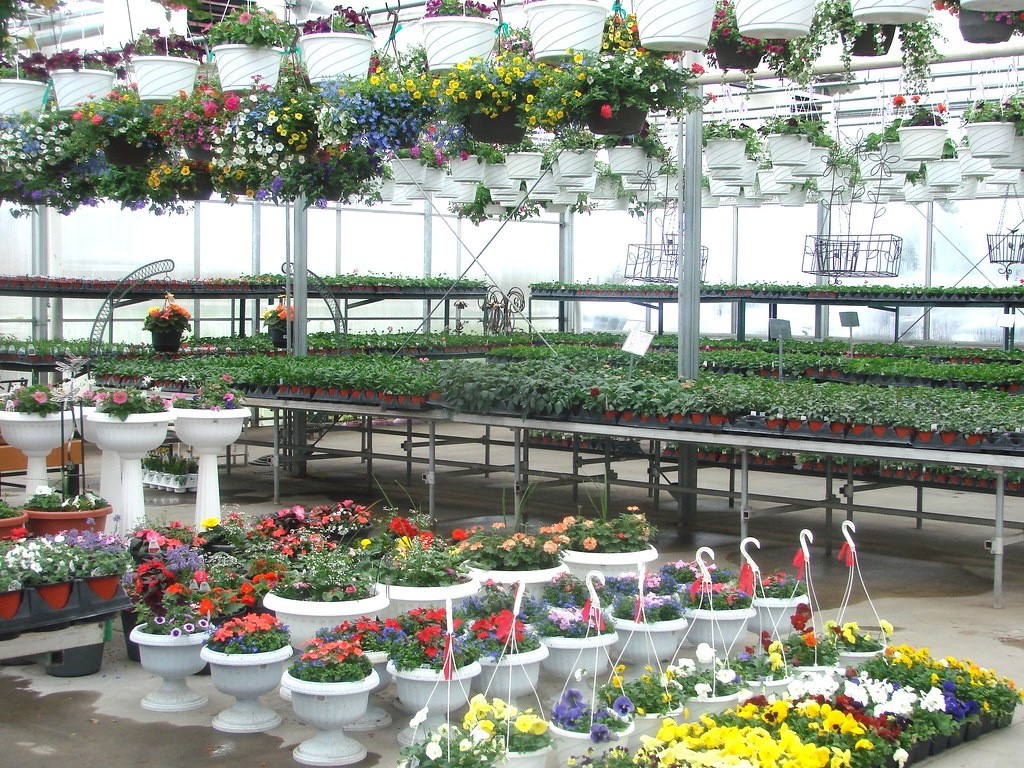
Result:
[452,414,1024,611]
[0,358,63,387]
[89,386,456,532]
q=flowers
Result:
[0,275,1024,501]
[0,484,1024,768]
[0,0,1024,238]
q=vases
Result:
[0,0,1012,767]
